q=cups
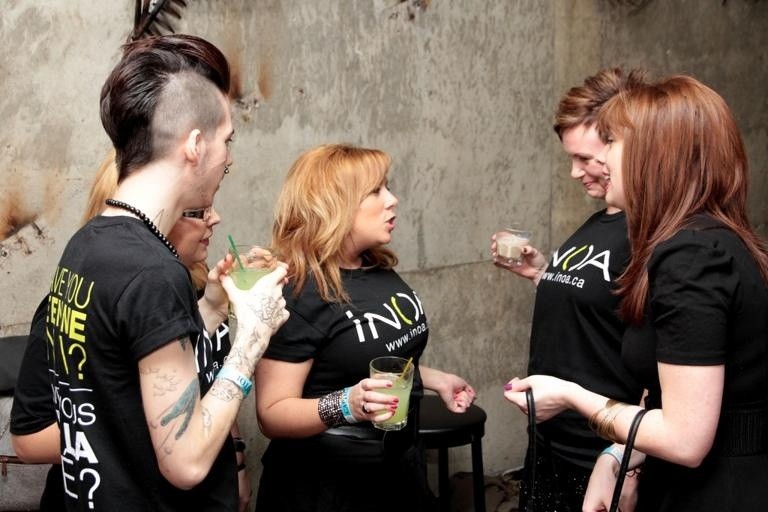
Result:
[492,213,532,271]
[365,355,415,435]
[226,244,279,342]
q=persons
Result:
[491,69,646,511]
[43,33,291,511]
[11,148,254,511]
[254,144,478,512]
[502,73,766,511]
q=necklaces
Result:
[105,198,181,259]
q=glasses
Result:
[183,207,212,221]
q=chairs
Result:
[0,336,87,508]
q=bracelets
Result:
[236,461,246,474]
[599,448,624,469]
[218,367,253,399]
[317,390,348,431]
[233,435,247,451]
[340,385,356,425]
[588,399,626,442]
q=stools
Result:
[419,393,486,511]
